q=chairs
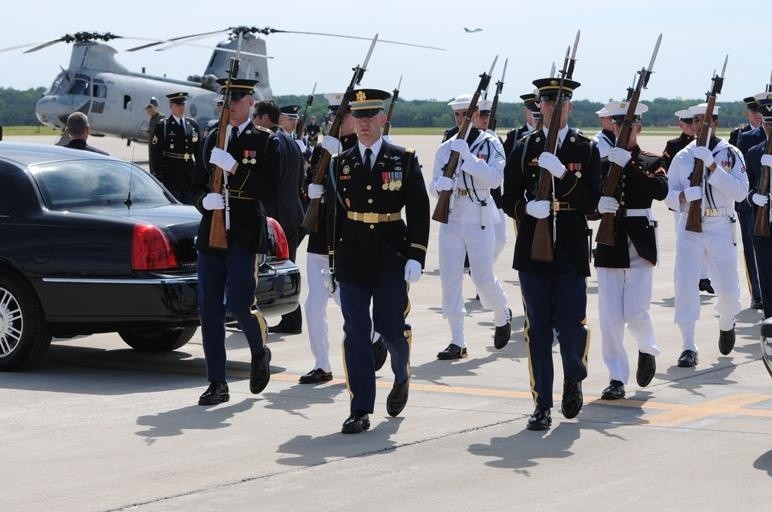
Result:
[71,86,84,95]
[92,174,121,195]
[86,85,100,96]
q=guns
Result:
[529,29,581,262]
[382,76,403,136]
[208,31,244,248]
[300,33,380,231]
[752,71,771,240]
[432,53,509,223]
[297,81,320,140]
[596,33,662,248]
[684,54,729,234]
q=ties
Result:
[180,119,184,134]
[364,149,372,174]
[231,126,238,146]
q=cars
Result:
[0,141,302,373]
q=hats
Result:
[744,92,772,123]
[675,103,720,123]
[448,95,492,113]
[214,79,258,106]
[595,102,648,124]
[520,78,580,120]
[324,89,390,117]
[166,92,188,104]
[280,105,300,120]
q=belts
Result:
[347,211,401,223]
[624,208,652,217]
[165,152,191,162]
[456,189,485,197]
[705,208,734,217]
[229,189,256,200]
[552,202,575,210]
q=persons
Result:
[145,78,771,435]
[64,112,109,155]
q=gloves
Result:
[403,259,421,283]
[202,193,225,210]
[527,199,551,219]
[308,183,324,200]
[684,147,772,206]
[437,139,471,191]
[209,146,236,172]
[538,152,565,179]
[597,196,619,214]
[608,146,631,168]
[321,136,343,156]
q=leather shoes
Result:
[249,347,270,394]
[751,299,763,309]
[527,352,655,431]
[678,323,735,367]
[342,336,409,433]
[268,322,301,335]
[495,309,511,349]
[198,381,230,406]
[699,279,714,293]
[437,344,467,360]
[299,369,332,384]
